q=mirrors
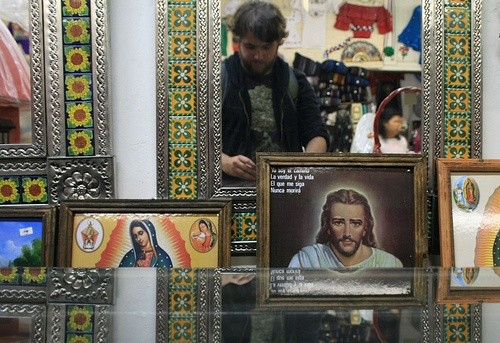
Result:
[0,0,116,207]
[154,0,483,257]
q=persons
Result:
[378,108,416,154]
[220,1,329,182]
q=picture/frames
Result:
[255,151,429,310]
[436,158,500,302]
[1,206,57,267]
[56,198,234,267]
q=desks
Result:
[343,60,422,118]
